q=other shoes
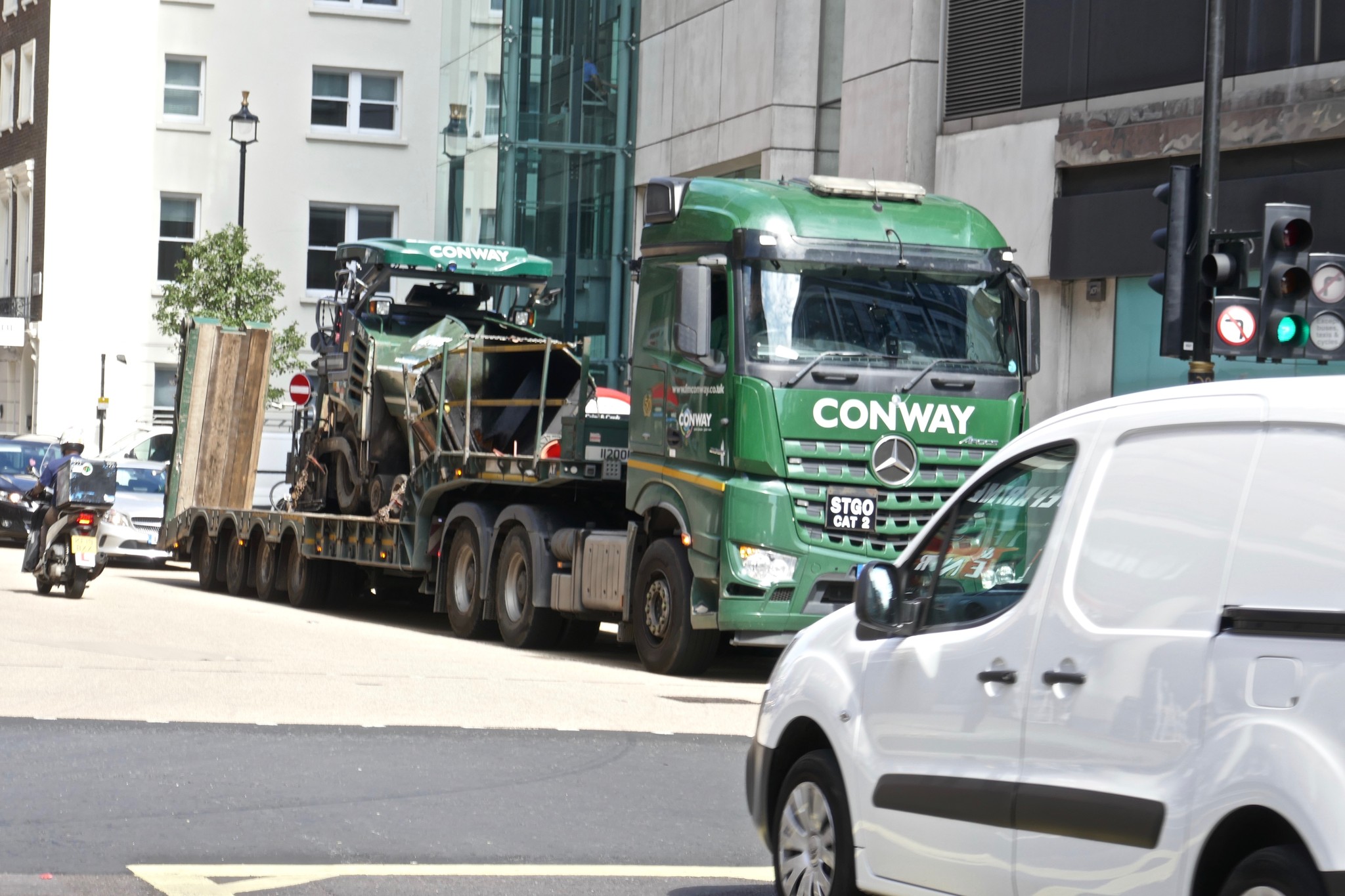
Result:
[34,559,43,570]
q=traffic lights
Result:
[1201,239,1251,294]
[1147,164,1202,361]
[1257,202,1314,359]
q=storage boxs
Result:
[57,456,117,511]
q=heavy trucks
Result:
[175,169,1045,679]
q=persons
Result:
[583,54,600,115]
[710,282,766,363]
[22,429,85,570]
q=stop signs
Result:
[289,372,311,406]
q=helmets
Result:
[60,429,84,455]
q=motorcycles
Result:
[19,459,118,599]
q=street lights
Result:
[228,90,259,330]
[441,103,469,243]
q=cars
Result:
[739,374,1345,896]
[0,423,174,567]
